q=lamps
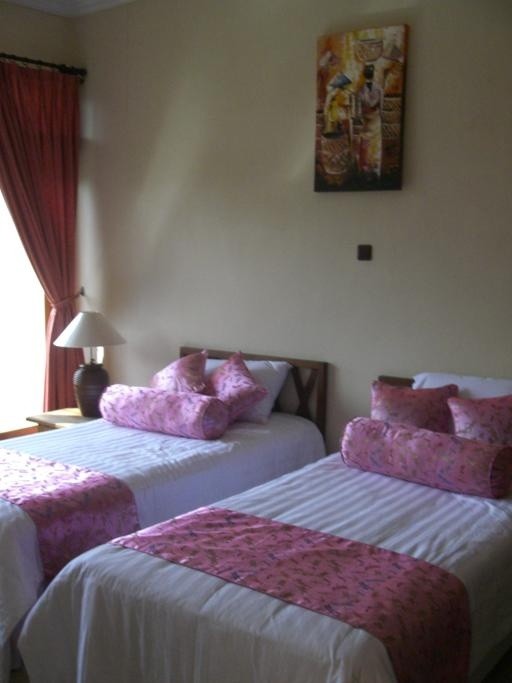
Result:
[52,312,127,419]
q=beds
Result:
[0,346,329,646]
[19,374,512,682]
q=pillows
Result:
[203,358,292,426]
[446,393,511,491]
[339,416,509,498]
[204,350,269,422]
[149,350,209,392]
[411,371,510,397]
[99,383,230,439]
[368,378,459,433]
